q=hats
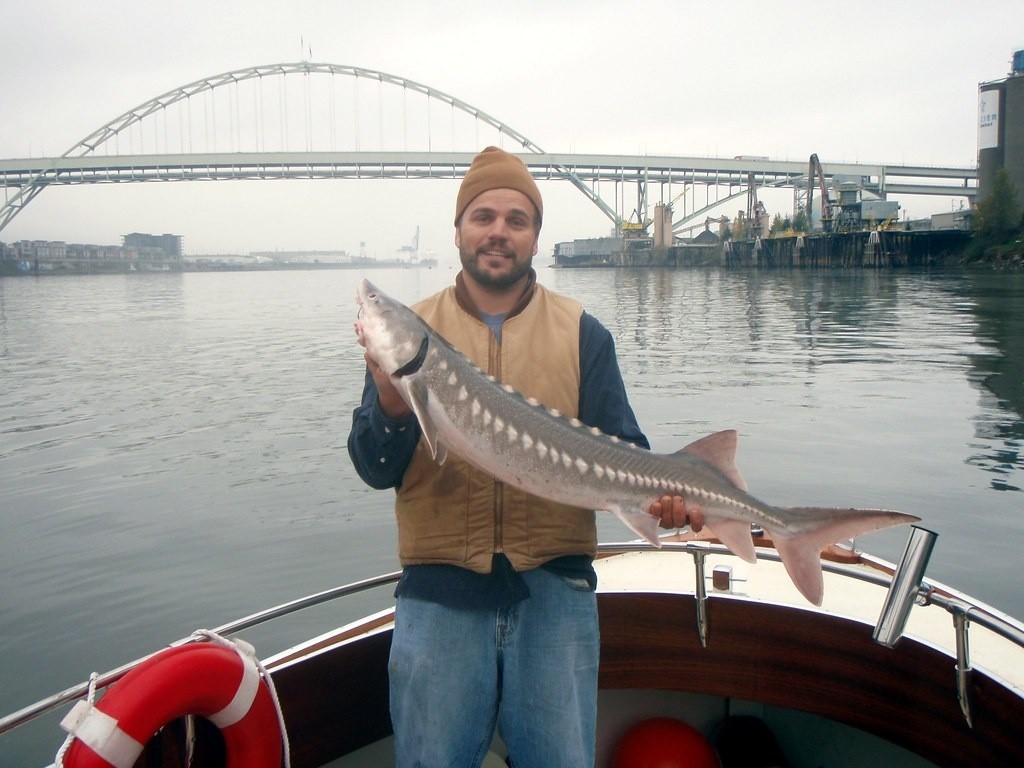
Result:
[454,146,543,228]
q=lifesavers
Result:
[55,642,284,768]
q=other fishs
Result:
[352,274,923,607]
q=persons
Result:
[347,147,703,768]
